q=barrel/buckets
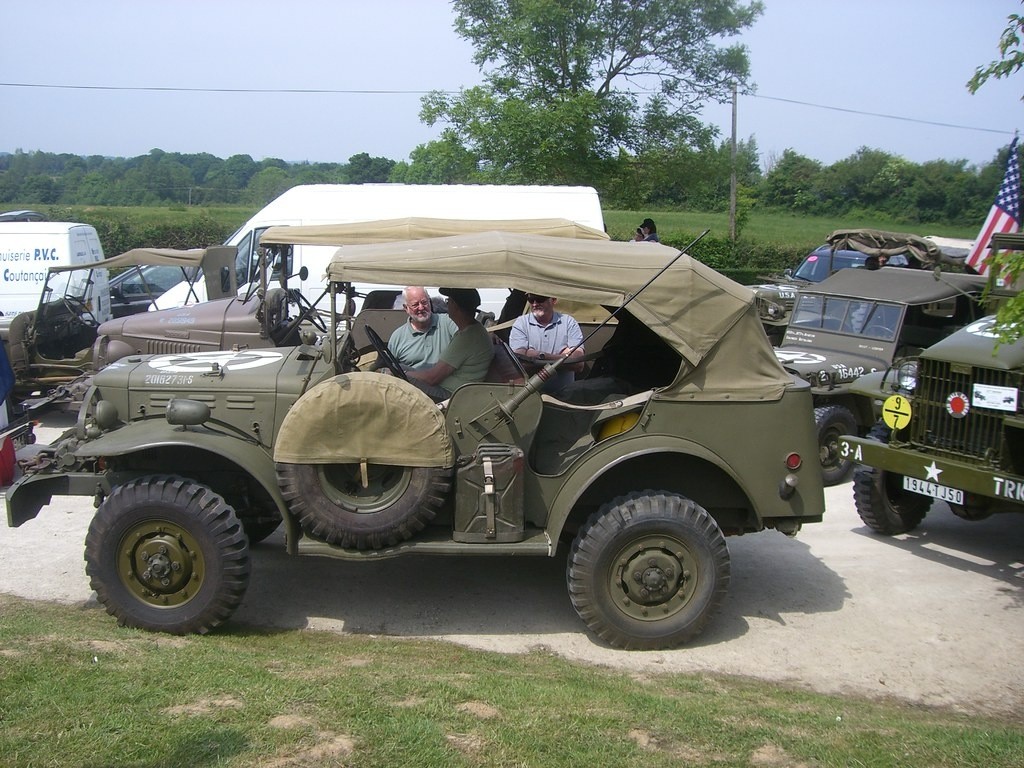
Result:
[452,444,524,543]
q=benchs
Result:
[482,343,528,383]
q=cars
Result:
[0,210,48,223]
[107,252,291,322]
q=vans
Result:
[148,183,604,349]
[0,221,115,343]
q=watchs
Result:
[538,351,546,361]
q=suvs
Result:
[5,215,1006,653]
[838,235,1024,534]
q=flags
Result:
[964,136,1024,293]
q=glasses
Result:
[527,295,552,304]
[444,298,459,308]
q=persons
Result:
[865,252,890,270]
[370,285,496,403]
[628,218,658,243]
[509,292,586,395]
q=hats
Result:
[637,228,645,236]
[439,287,481,308]
[639,218,655,228]
